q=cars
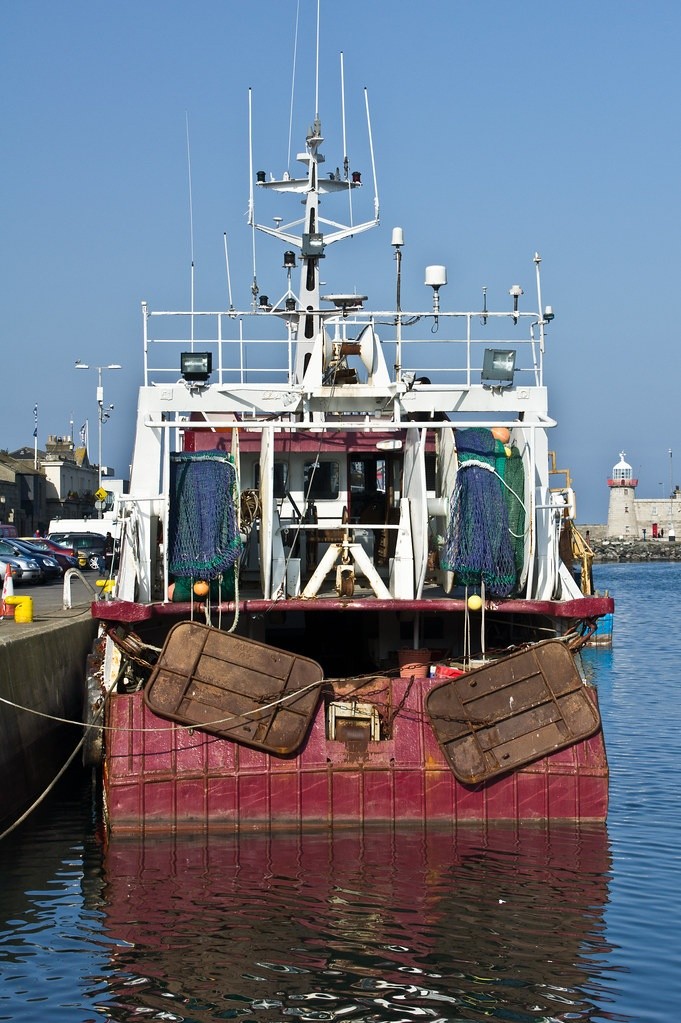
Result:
[0,524,121,583]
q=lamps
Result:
[180,352,212,382]
[480,346,517,381]
[302,233,324,255]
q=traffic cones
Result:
[0,562,17,620]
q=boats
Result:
[56,0,624,840]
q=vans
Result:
[47,518,122,562]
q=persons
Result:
[102,532,119,576]
[98,551,105,577]
[33,529,40,538]
[44,531,48,538]
[653,528,664,538]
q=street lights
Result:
[74,364,122,519]
